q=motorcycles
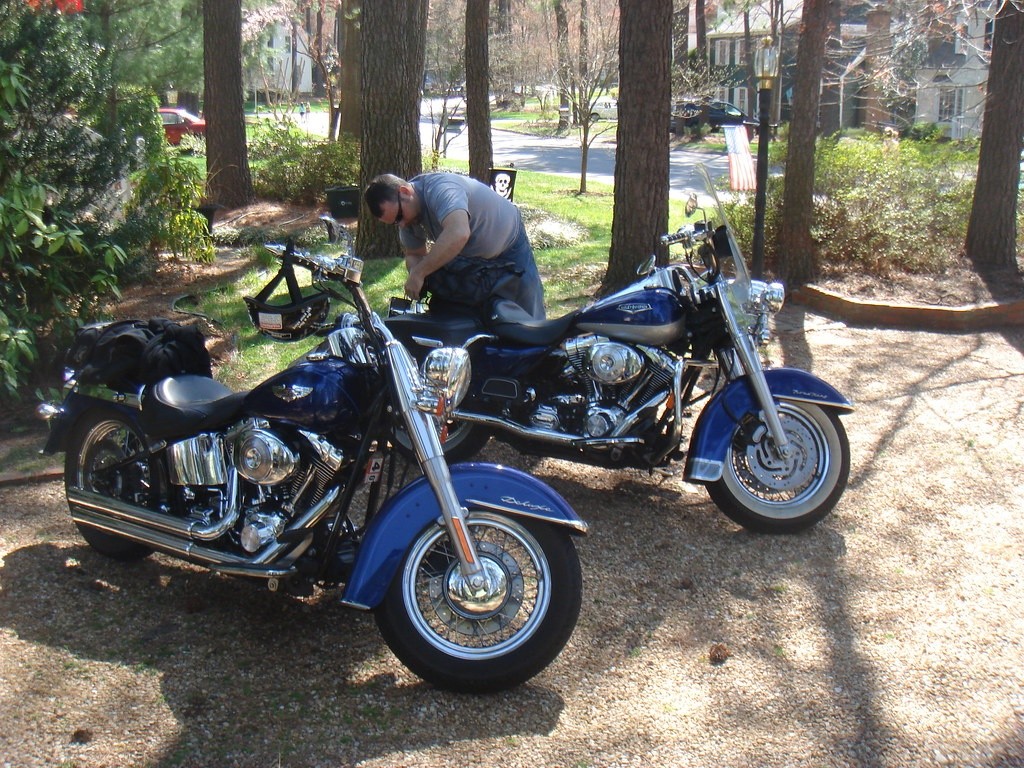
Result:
[35,215,589,699]
[366,194,855,540]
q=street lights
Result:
[750,33,780,287]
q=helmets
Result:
[244,293,331,342]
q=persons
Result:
[299,101,311,123]
[363,172,548,325]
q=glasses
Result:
[391,192,404,226]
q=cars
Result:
[671,96,761,139]
[588,99,618,123]
[155,108,206,146]
